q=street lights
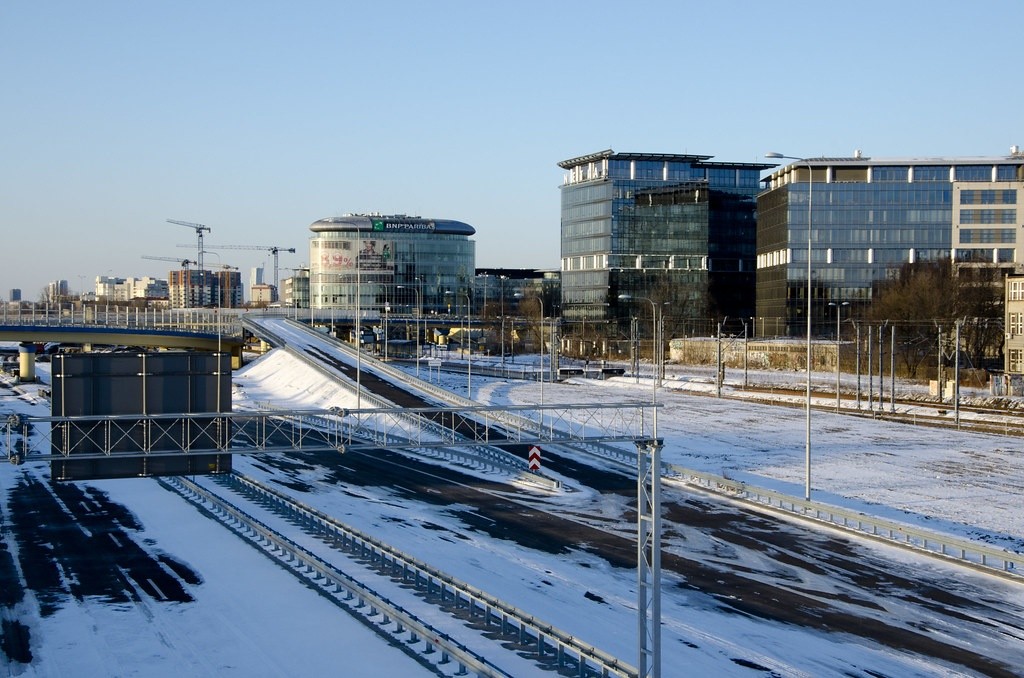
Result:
[496,315,510,369]
[514,293,543,423]
[420,315,431,357]
[547,316,561,382]
[766,152,811,501]
[652,302,670,388]
[619,295,656,404]
[445,291,471,399]
[828,302,849,413]
[367,280,387,363]
[455,315,468,360]
[324,218,360,437]
[397,286,419,378]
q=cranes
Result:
[142,254,196,309]
[167,218,211,308]
[176,245,296,302]
